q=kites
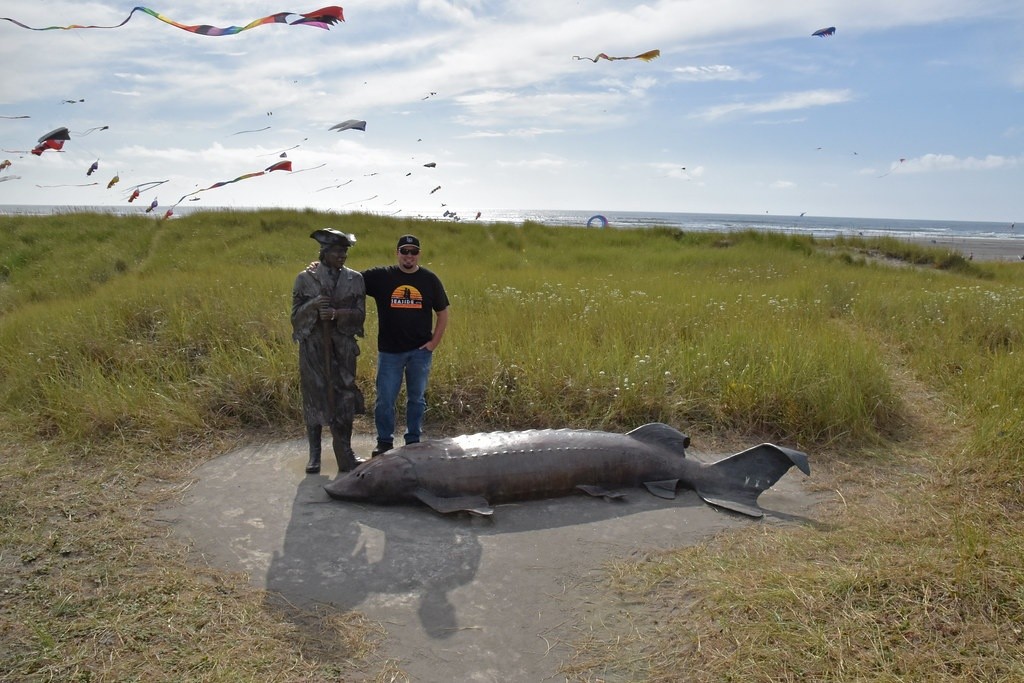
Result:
[811,26,838,38]
[0,92,482,226]
[571,49,661,63]
[0,6,343,37]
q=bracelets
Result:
[332,309,335,320]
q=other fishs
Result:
[323,422,810,518]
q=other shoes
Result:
[372,443,393,457]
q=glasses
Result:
[399,248,419,255]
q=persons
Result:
[290,226,367,474]
[303,235,451,459]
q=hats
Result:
[397,235,420,249]
